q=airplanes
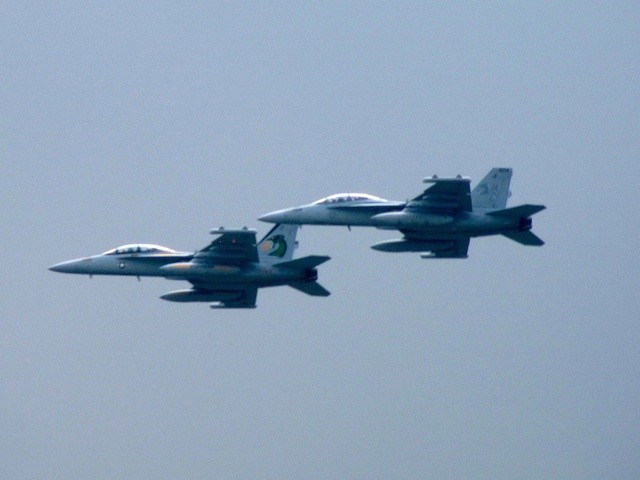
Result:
[256,166,545,258]
[49,224,330,308]
